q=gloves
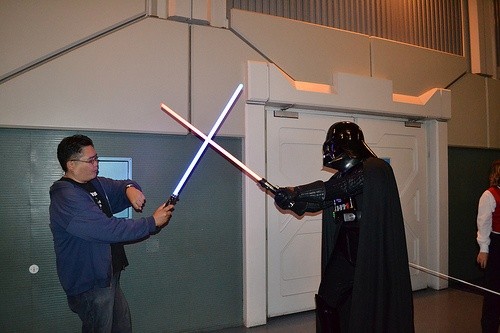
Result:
[279,199,321,216]
[275,180,326,205]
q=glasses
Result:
[68,156,98,164]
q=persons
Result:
[476,160,500,333]
[272,119,400,333]
[45,133,176,333]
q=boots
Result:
[315,293,349,333]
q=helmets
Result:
[322,121,364,150]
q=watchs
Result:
[125,184,134,188]
